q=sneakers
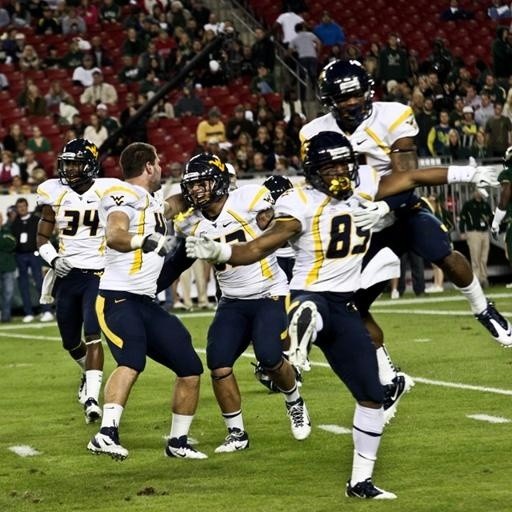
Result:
[390,285,444,300]
[213,425,251,456]
[283,395,313,440]
[81,396,104,425]
[22,314,34,323]
[473,299,512,347]
[86,427,130,461]
[162,435,208,461]
[285,300,319,369]
[39,312,54,322]
[380,370,416,429]
[344,476,398,500]
[74,378,87,405]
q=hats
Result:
[463,106,473,113]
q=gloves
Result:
[447,156,500,198]
[128,232,173,257]
[38,240,74,279]
[183,232,232,265]
[354,197,392,232]
[490,207,506,241]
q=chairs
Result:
[0,1,512,179]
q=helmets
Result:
[501,146,512,168]
[179,151,230,211]
[318,57,376,124]
[302,130,362,200]
[262,173,293,201]
[57,137,102,186]
